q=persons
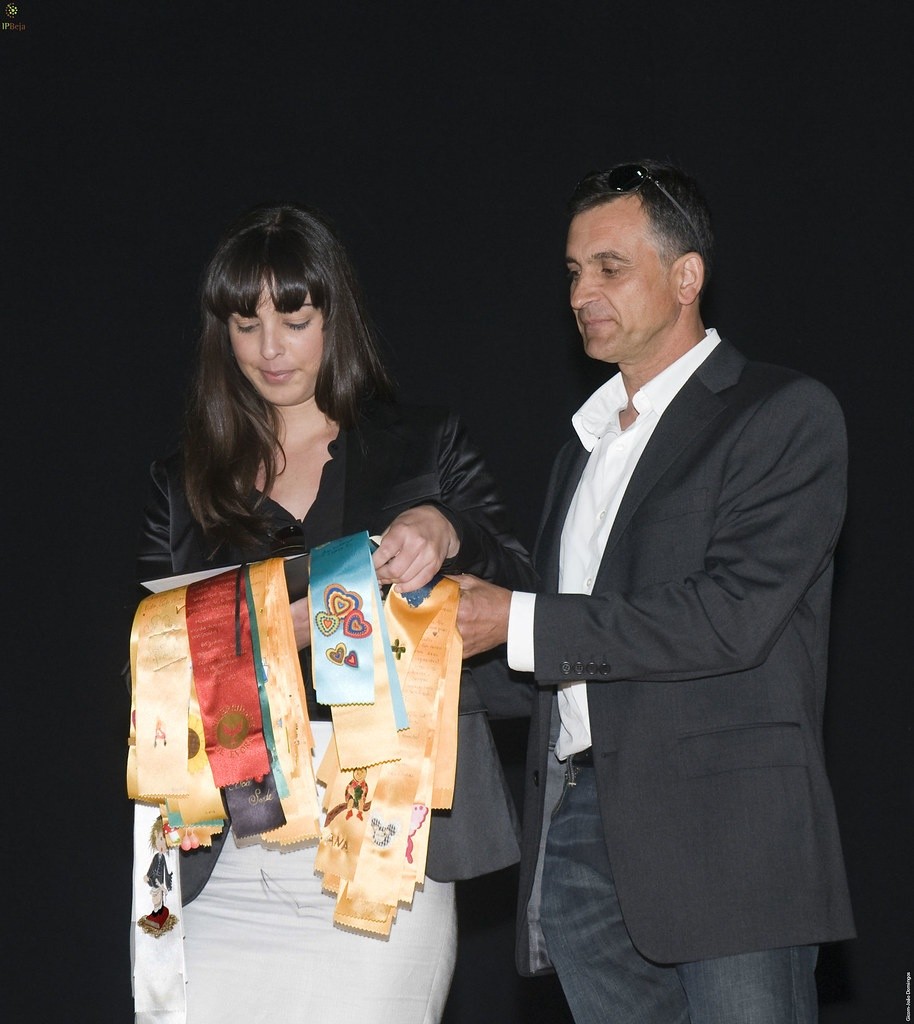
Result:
[446,164,855,1024]
[112,205,539,1024]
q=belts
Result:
[572,747,593,768]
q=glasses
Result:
[570,163,704,260]
